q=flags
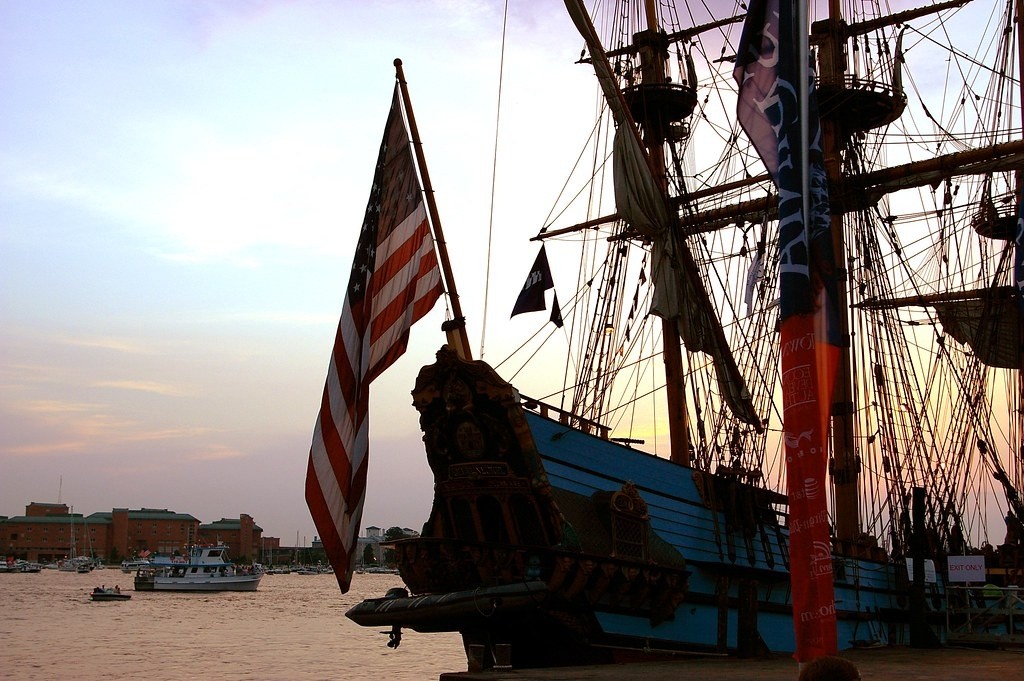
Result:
[549,292,563,328]
[632,284,639,311]
[745,253,763,317]
[305,80,448,595]
[510,245,555,319]
[639,269,647,286]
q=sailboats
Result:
[347,2,1024,670]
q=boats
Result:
[133,563,261,591]
[0,505,105,574]
[120,539,400,578]
[89,587,131,601]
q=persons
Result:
[113,584,120,594]
[101,585,106,592]
